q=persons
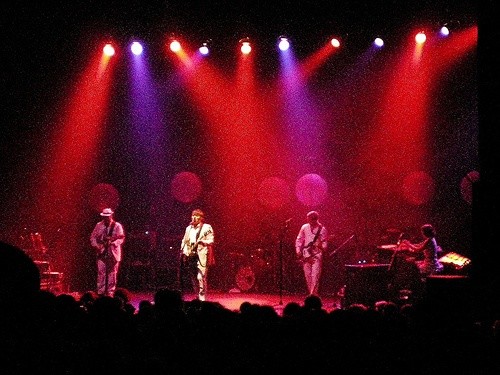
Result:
[0,245,500,375]
[181,209,214,302]
[295,210,326,298]
[403,224,443,275]
[90,208,124,299]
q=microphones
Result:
[104,219,109,227]
[194,217,197,227]
[346,235,355,242]
[281,218,294,225]
[397,233,405,245]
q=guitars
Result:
[97,235,124,255]
[191,229,211,252]
[302,234,334,258]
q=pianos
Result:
[377,244,442,251]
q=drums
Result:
[227,253,244,272]
[235,267,262,290]
[250,248,264,267]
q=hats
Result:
[100,208,114,216]
